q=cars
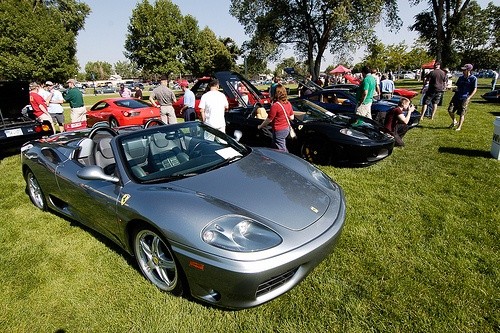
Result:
[95,87,116,94]
[118,80,145,91]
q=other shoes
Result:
[450,122,459,127]
[455,128,461,131]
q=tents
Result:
[329,65,351,73]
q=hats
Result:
[461,64,473,71]
[444,67,450,72]
[434,61,441,66]
[46,81,53,85]
[161,76,167,80]
[120,84,124,88]
[361,66,370,73]
[66,79,75,83]
[179,80,189,86]
[29,82,39,87]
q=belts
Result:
[71,106,84,108]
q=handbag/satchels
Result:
[290,127,296,138]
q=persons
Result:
[420,62,449,120]
[179,81,196,122]
[29,80,64,135]
[448,64,477,131]
[119,84,131,97]
[237,82,250,107]
[317,76,335,87]
[134,86,142,99]
[380,73,395,99]
[149,75,178,125]
[491,73,498,91]
[198,79,229,144]
[270,79,279,98]
[371,69,380,100]
[63,78,86,128]
[384,97,415,146]
[355,66,376,119]
[257,84,295,153]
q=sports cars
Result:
[0,80,54,151]
[20,122,347,311]
[156,67,421,167]
[85,98,161,132]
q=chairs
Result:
[79,126,148,179]
[144,119,192,173]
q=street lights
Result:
[241,41,250,81]
